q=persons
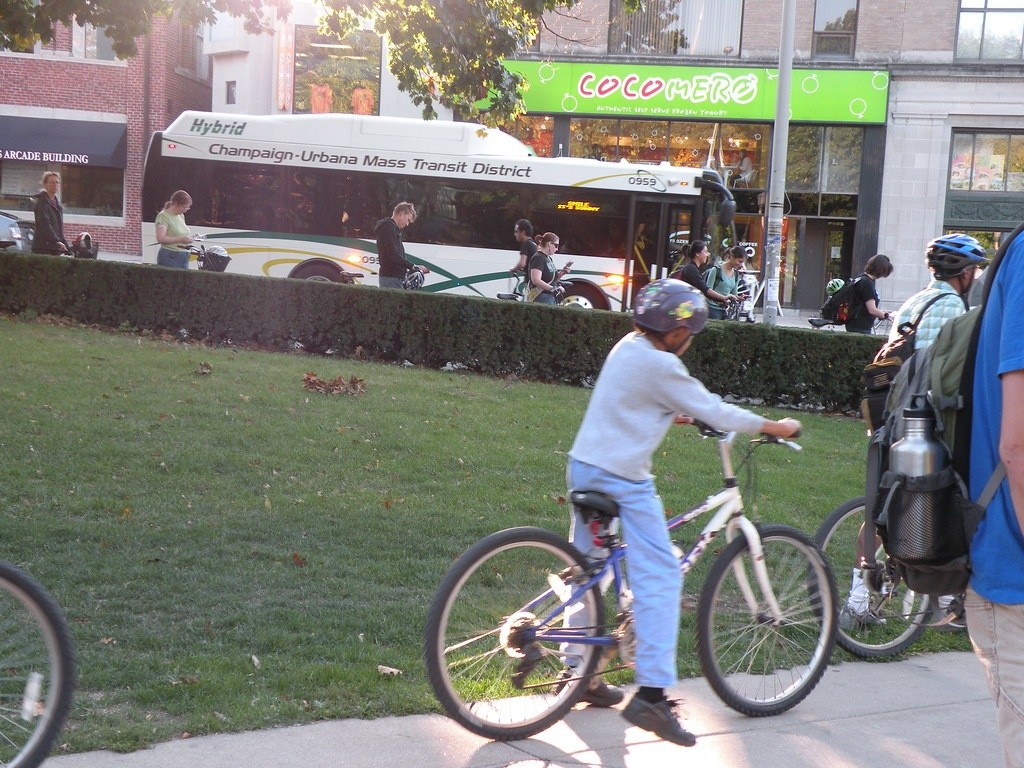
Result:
[703,246,750,320]
[510,219,538,286]
[155,190,194,269]
[964,227,1024,768]
[374,201,428,289]
[846,254,896,336]
[729,148,753,188]
[31,170,69,255]
[525,232,570,304]
[839,234,987,629]
[551,278,804,747]
[681,240,738,305]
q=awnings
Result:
[0,104,127,167]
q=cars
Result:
[0,209,35,254]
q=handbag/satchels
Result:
[526,283,540,303]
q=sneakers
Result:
[839,600,887,631]
[553,671,624,707]
[941,604,969,628]
[620,694,696,748]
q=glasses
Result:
[515,229,521,232]
[551,243,559,249]
[179,205,191,212]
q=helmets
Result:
[633,275,709,335]
[925,233,991,280]
[405,268,424,290]
[826,279,845,294]
[75,232,93,254]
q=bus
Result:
[140,108,739,311]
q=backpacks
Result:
[858,291,963,437]
[820,276,863,326]
[668,264,685,280]
[867,217,1024,594]
[700,266,738,291]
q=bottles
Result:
[671,538,690,564]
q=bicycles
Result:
[0,558,74,768]
[807,495,968,661]
[807,311,895,334]
[423,415,842,745]
[143,244,232,273]
[0,239,100,260]
[497,285,568,306]
[307,266,428,290]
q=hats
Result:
[207,245,229,258]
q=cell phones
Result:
[194,239,201,242]
[564,261,574,269]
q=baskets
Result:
[71,240,99,260]
[202,254,231,272]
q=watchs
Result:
[881,313,889,319]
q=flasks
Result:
[886,387,949,564]
